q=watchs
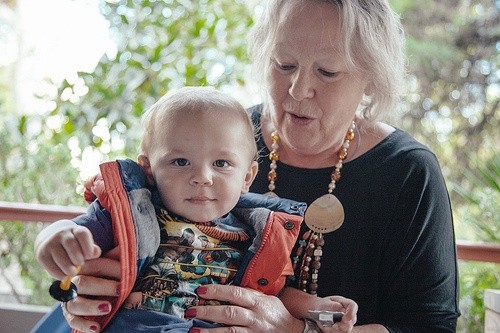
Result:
[302,318,321,333]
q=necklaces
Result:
[268,115,361,297]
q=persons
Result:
[34,87,358,333]
[59,0,461,333]
